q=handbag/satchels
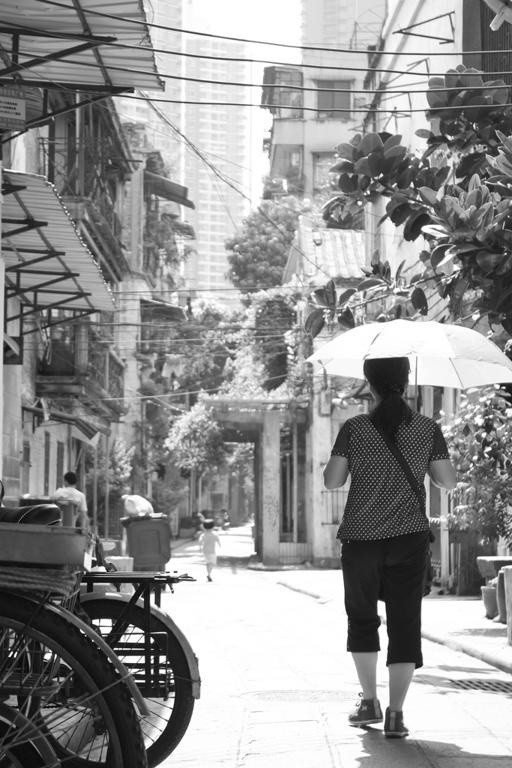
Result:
[378,531,436,602]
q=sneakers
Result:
[349,691,382,725]
[384,706,408,738]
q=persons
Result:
[323,358,456,739]
[51,472,88,513]
[199,519,220,582]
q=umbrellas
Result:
[303,318,512,412]
